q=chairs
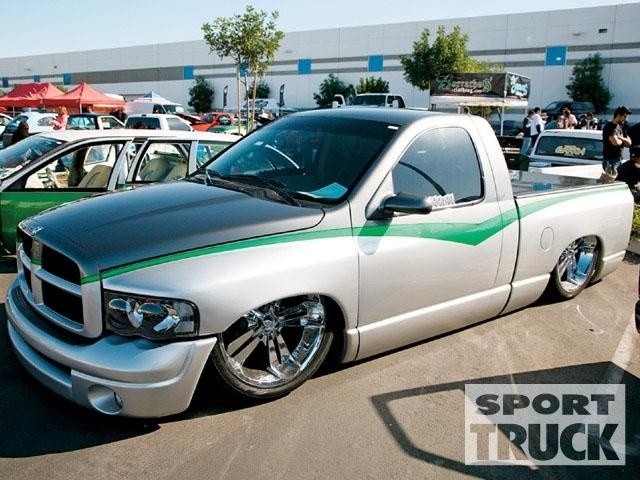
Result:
[76,158,186,188]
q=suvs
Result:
[522,127,633,188]
[493,120,526,138]
[541,98,596,125]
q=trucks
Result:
[329,90,429,111]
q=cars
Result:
[0,129,301,267]
[0,94,298,152]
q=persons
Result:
[48,104,68,130]
[10,121,30,145]
[602,105,633,175]
[518,105,599,157]
[615,145,640,205]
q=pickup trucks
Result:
[0,103,634,421]
